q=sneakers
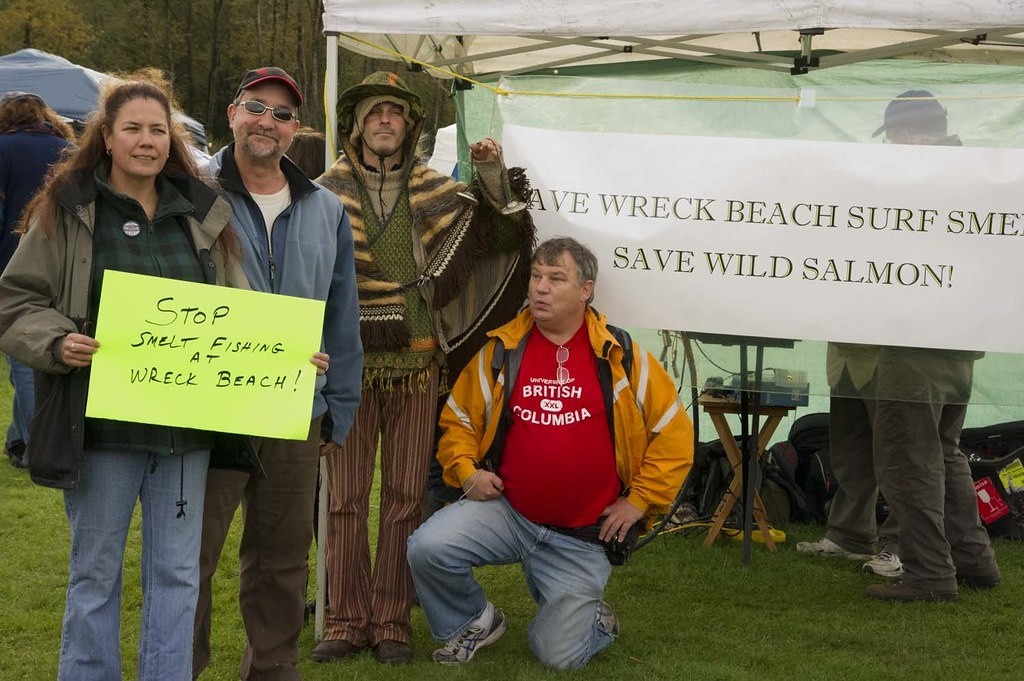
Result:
[796,538,876,560]
[862,551,905,576]
[595,600,619,638]
[431,601,506,665]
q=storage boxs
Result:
[733,381,809,408]
[733,367,809,388]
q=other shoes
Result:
[378,638,410,663]
[868,582,957,602]
[310,639,364,661]
[955,570,1001,588]
[4,440,29,466]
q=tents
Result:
[1,46,212,158]
[310,3,1024,184]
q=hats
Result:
[872,91,942,136]
[237,67,303,105]
[349,96,415,146]
[0,91,46,110]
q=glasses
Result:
[237,100,297,121]
[556,345,570,388]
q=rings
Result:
[71,342,75,351]
[326,363,330,371]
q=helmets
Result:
[336,70,423,130]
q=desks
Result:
[695,384,798,552]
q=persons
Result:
[0,80,335,681]
[308,74,517,664]
[408,237,695,669]
[193,68,368,681]
[795,90,999,602]
[1,88,88,470]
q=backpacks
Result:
[675,413,889,528]
